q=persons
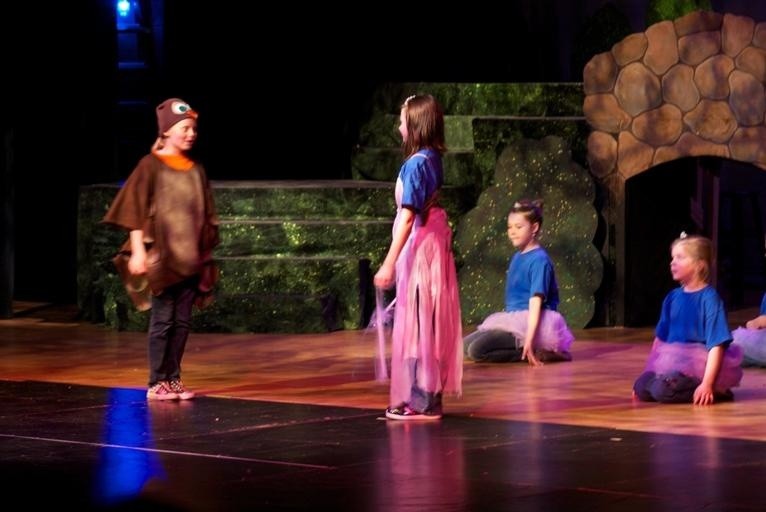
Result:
[371,92,464,422]
[101,96,220,401]
[632,229,743,407]
[729,291,765,371]
[460,196,574,367]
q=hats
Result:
[156,99,200,137]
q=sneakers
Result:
[147,379,195,400]
[385,405,443,421]
[545,347,572,361]
